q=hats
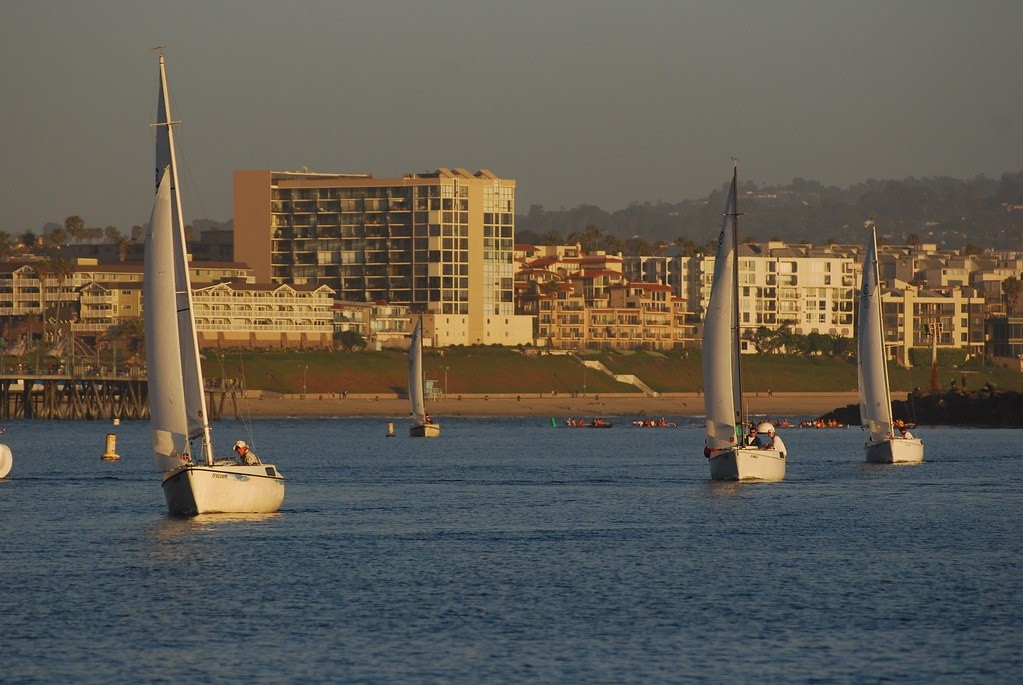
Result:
[233,440,249,452]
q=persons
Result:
[7,362,148,378]
[765,428,787,456]
[633,417,668,427]
[232,441,258,466]
[566,416,606,427]
[746,417,845,428]
[893,419,905,428]
[204,376,241,389]
[425,412,432,424]
[900,427,914,439]
[739,427,763,449]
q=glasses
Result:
[750,431,756,434]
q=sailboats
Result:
[856,225,924,465]
[697,151,793,486]
[130,37,287,517]
[409,315,440,437]
